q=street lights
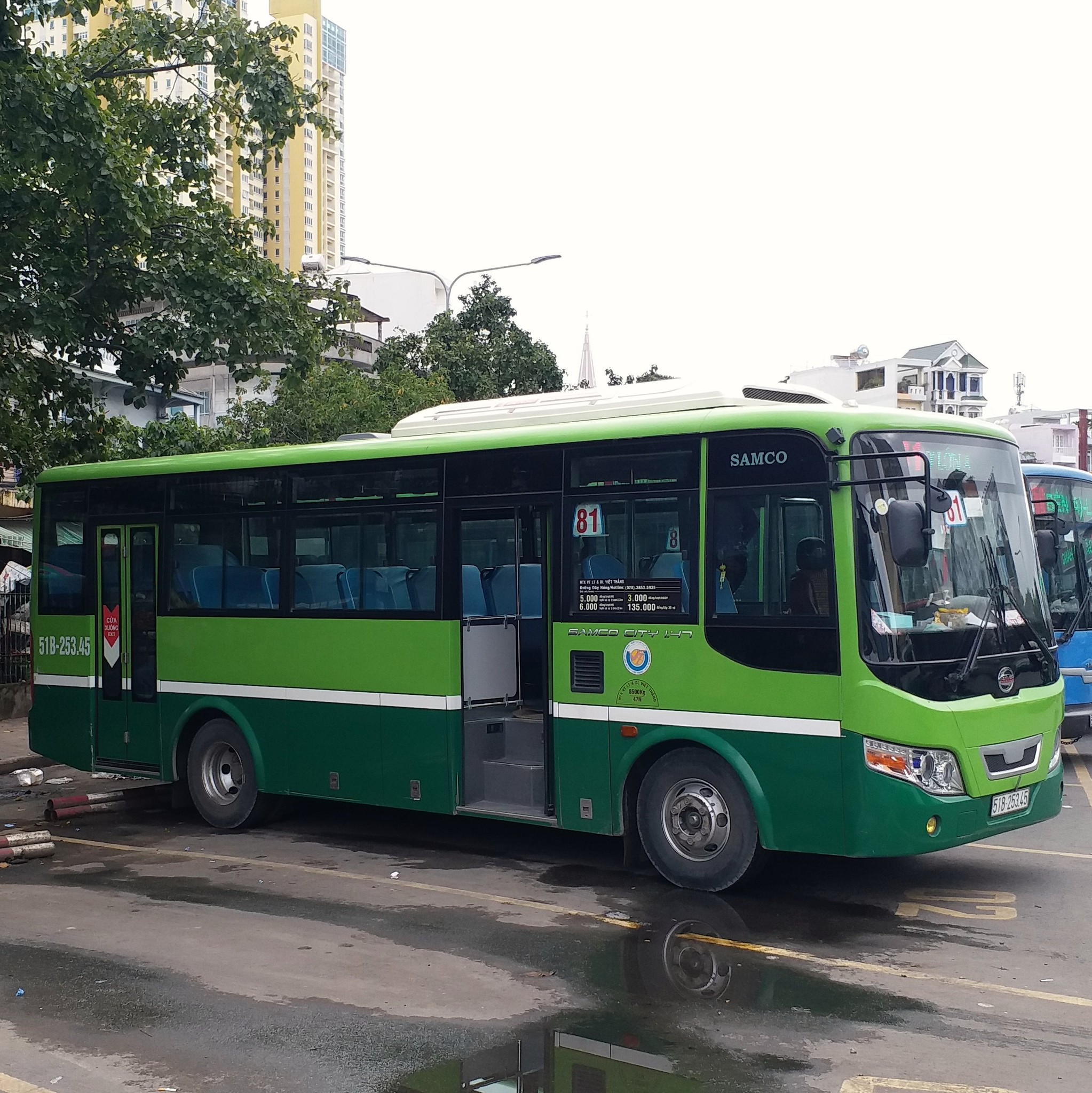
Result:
[341,253,563,321]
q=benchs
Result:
[47,543,739,619]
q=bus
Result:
[24,377,1062,896]
[1017,463,1091,741]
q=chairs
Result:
[791,534,828,617]
